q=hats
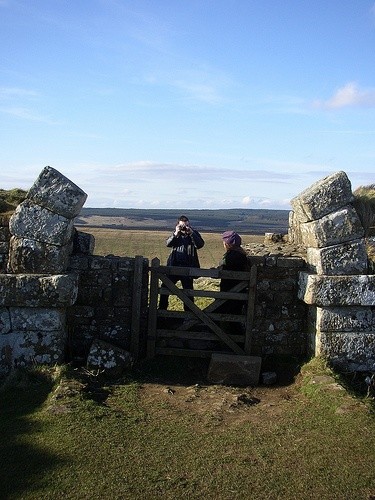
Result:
[223,230,242,248]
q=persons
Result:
[204,230,250,352]
[155,215,206,314]
